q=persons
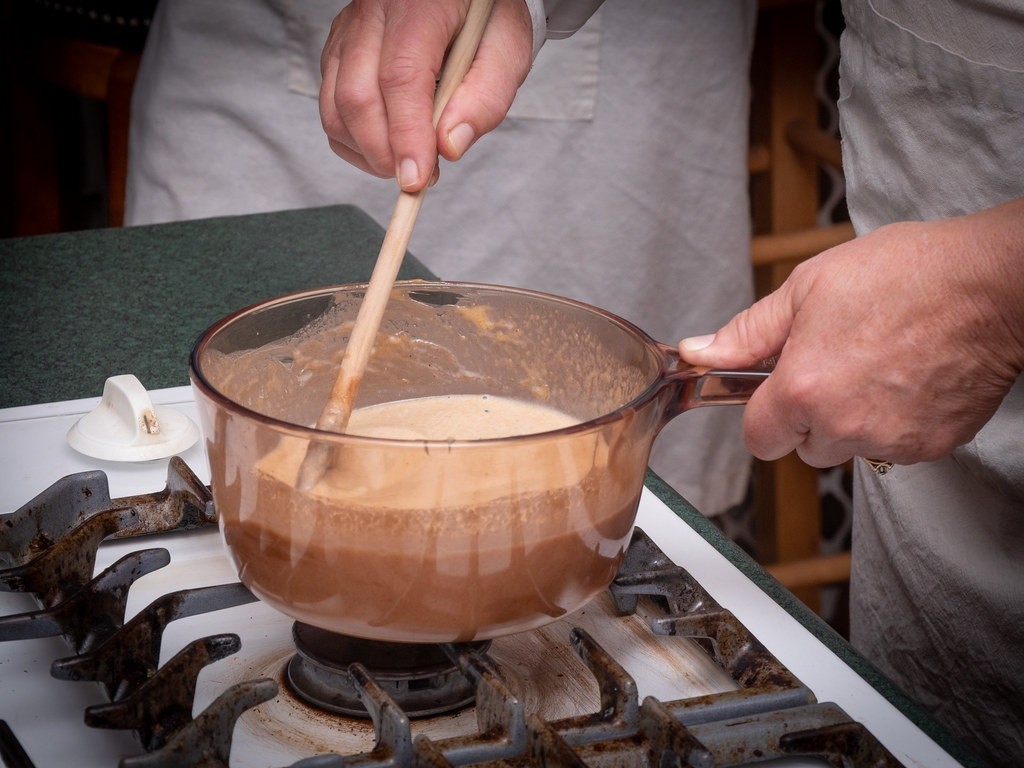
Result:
[122,0,1024,768]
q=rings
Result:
[862,457,894,477]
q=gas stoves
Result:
[0,374,965,768]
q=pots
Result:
[187,276,777,644]
